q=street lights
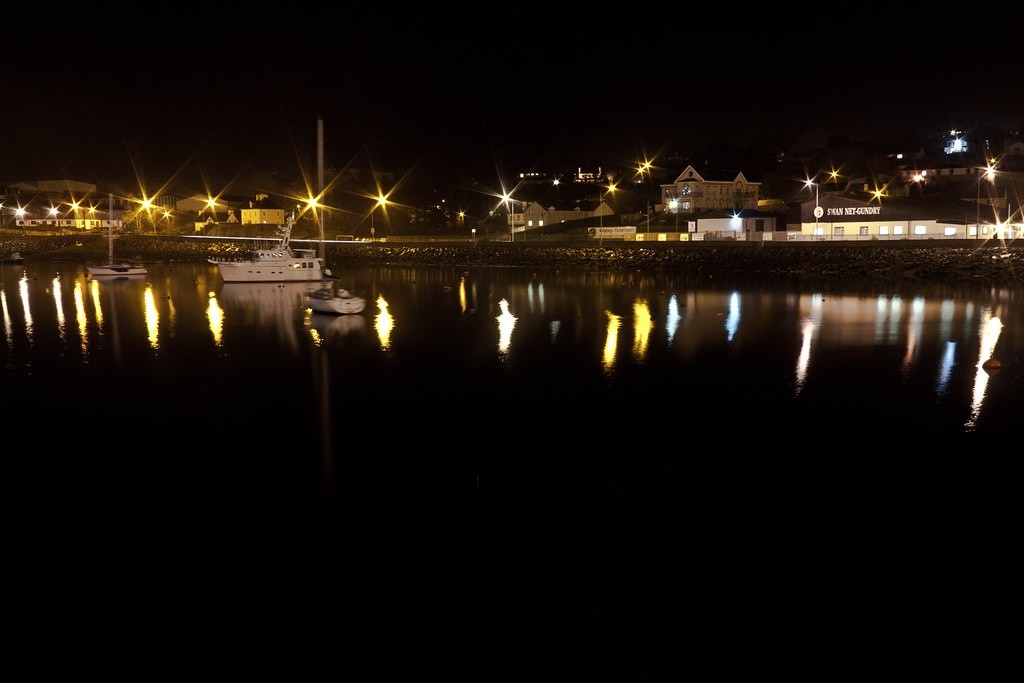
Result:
[504,195,514,242]
[807,181,819,241]
[976,166,992,238]
[600,184,614,237]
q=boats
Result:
[218,211,338,281]
[2,251,23,264]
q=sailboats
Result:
[308,120,367,315]
[85,193,148,274]
